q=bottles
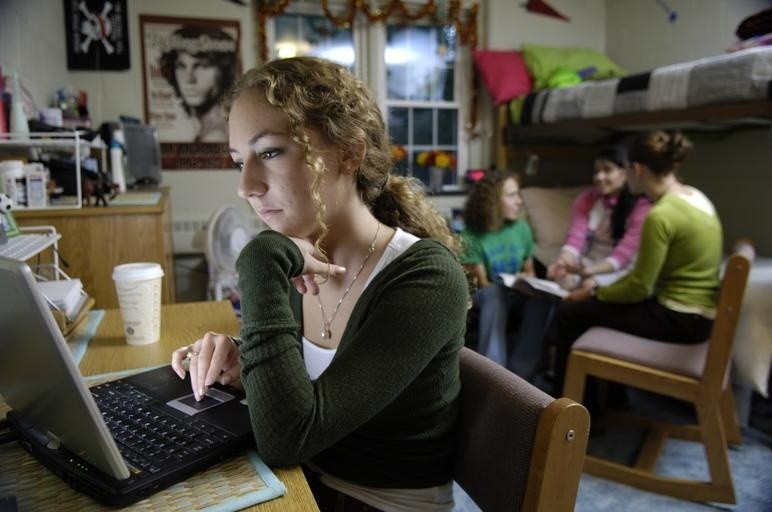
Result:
[111,142,129,194]
[54,86,88,119]
[27,163,47,207]
[9,69,30,138]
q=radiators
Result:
[173,212,273,275]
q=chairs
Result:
[560,235,757,505]
[454,343,591,511]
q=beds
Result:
[493,43,771,450]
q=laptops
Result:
[0,256,256,508]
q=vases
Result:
[429,166,446,195]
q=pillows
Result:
[521,41,632,92]
[520,181,590,249]
[474,49,530,106]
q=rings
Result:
[186,352,199,360]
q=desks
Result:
[10,185,174,308]
[0,298,324,512]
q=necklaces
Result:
[317,221,382,339]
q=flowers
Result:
[415,148,458,171]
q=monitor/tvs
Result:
[96,122,163,188]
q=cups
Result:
[1,160,27,204]
[112,262,165,345]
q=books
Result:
[38,279,106,368]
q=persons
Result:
[528,139,652,292]
[171,57,472,512]
[531,129,724,400]
[461,169,552,383]
[159,25,238,143]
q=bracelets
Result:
[589,286,598,300]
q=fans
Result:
[204,203,254,301]
